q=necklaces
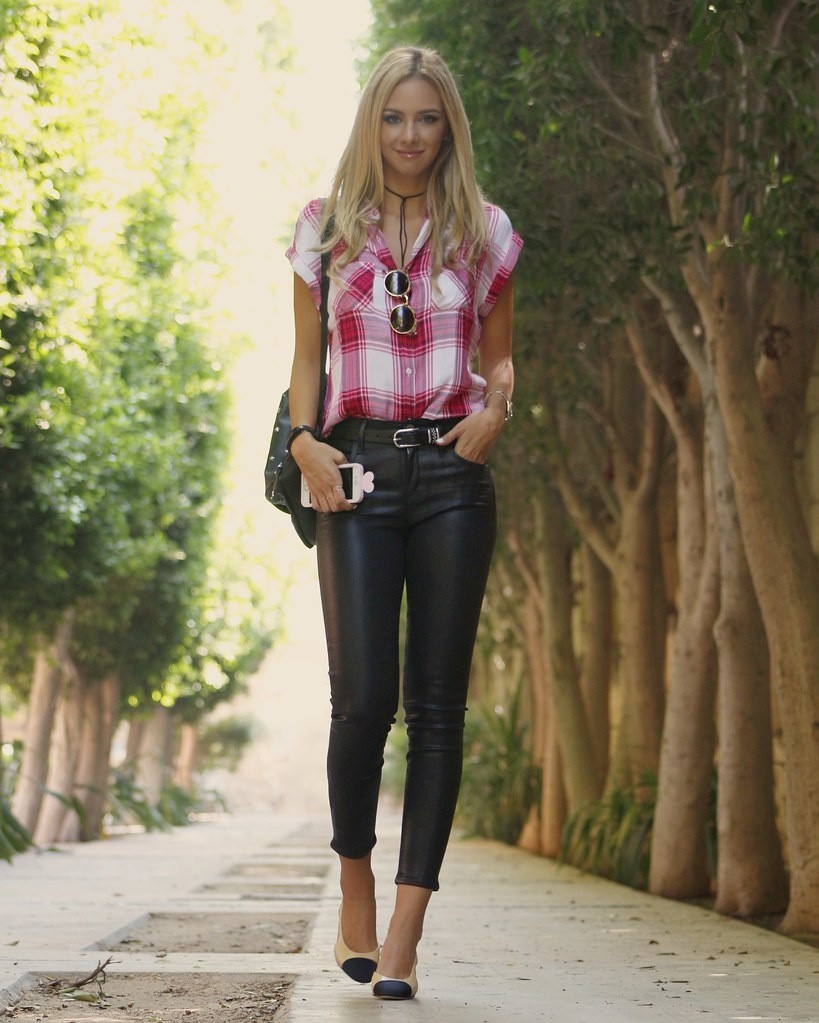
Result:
[385,185,427,270]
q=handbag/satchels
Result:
[263,373,328,548]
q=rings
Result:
[332,486,342,491]
[326,494,333,499]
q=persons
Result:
[286,47,523,1000]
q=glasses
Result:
[383,268,417,336]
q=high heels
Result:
[333,901,381,984]
[371,952,418,1000]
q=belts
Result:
[330,424,457,448]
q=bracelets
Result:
[483,391,513,420]
[286,425,314,448]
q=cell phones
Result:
[301,463,375,508]
